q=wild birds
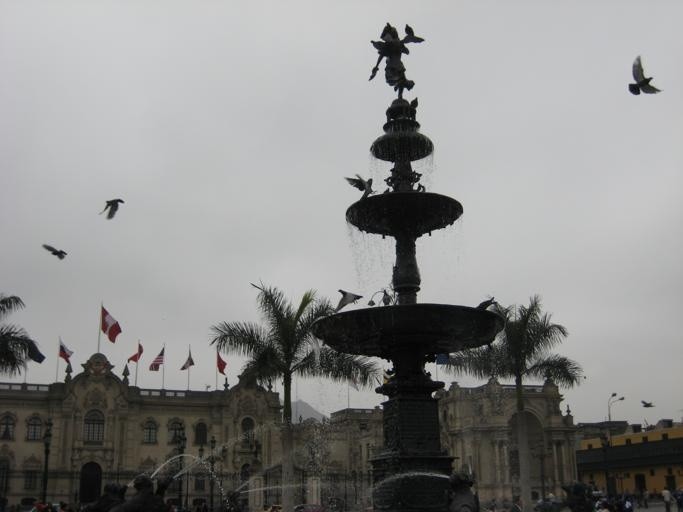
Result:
[370,40,384,49]
[386,22,392,30]
[42,243,68,260]
[405,24,415,37]
[98,198,125,220]
[337,289,363,303]
[641,400,655,408]
[411,97,418,108]
[382,289,391,306]
[475,296,498,309]
[628,55,662,96]
[343,173,378,201]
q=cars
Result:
[583,482,603,497]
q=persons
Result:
[366,24,418,95]
[475,482,683,512]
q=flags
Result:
[126,342,144,365]
[56,340,75,366]
[146,347,165,373]
[213,351,229,377]
[176,350,197,372]
[100,305,121,344]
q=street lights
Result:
[41,415,55,503]
[208,433,216,512]
[607,393,626,447]
[176,429,188,512]
[350,469,357,504]
